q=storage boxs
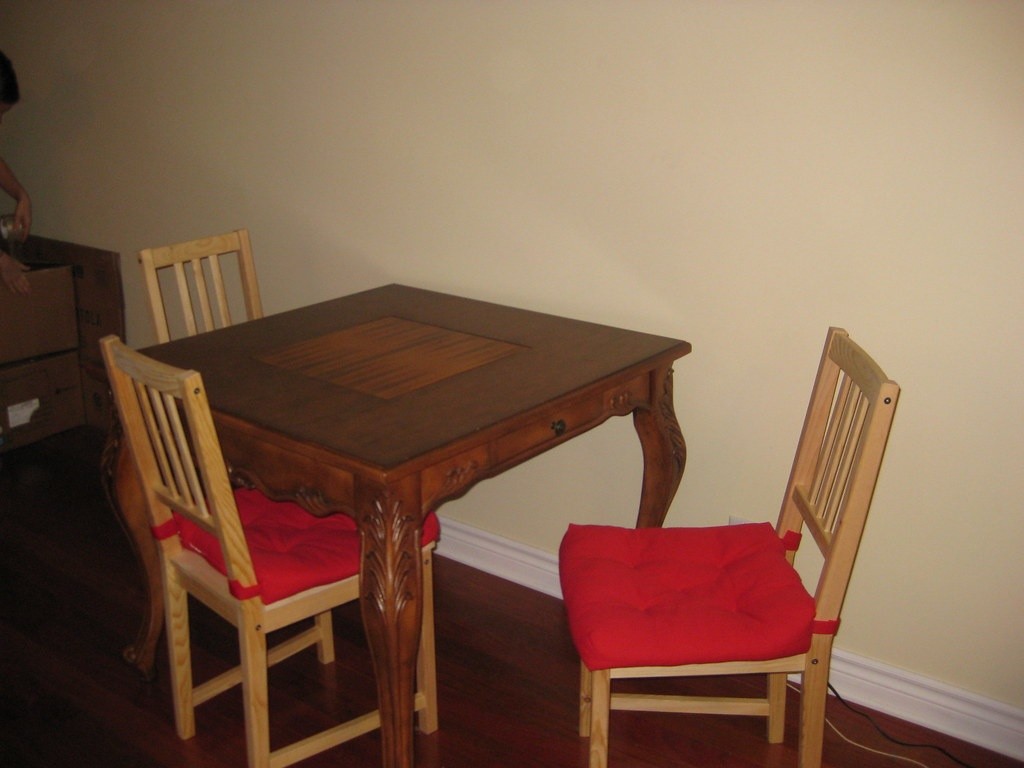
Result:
[0,349,89,454]
[0,259,82,365]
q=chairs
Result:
[135,227,264,344]
[558,326,901,768]
[97,333,438,768]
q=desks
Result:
[96,280,691,768]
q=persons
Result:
[0,52,33,301]
